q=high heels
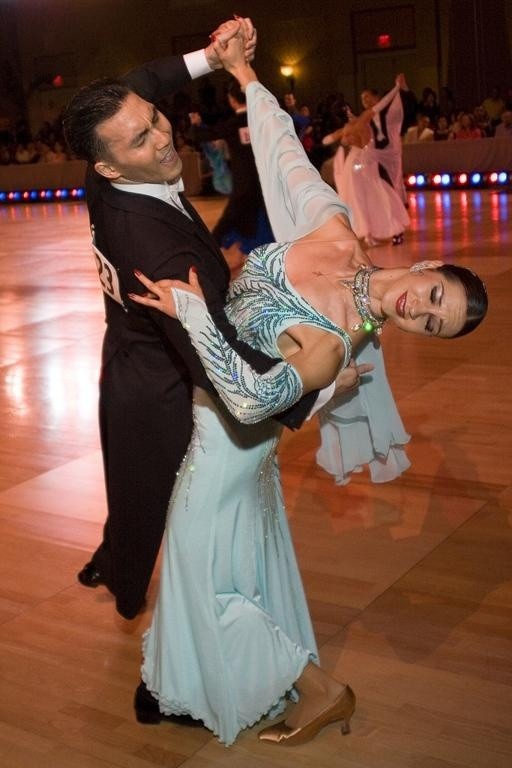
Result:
[257,685,355,746]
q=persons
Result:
[2,75,510,249]
[51,13,372,626]
[121,17,489,749]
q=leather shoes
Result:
[77,561,141,620]
[135,681,204,727]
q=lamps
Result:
[277,63,298,91]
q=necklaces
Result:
[311,261,384,336]
